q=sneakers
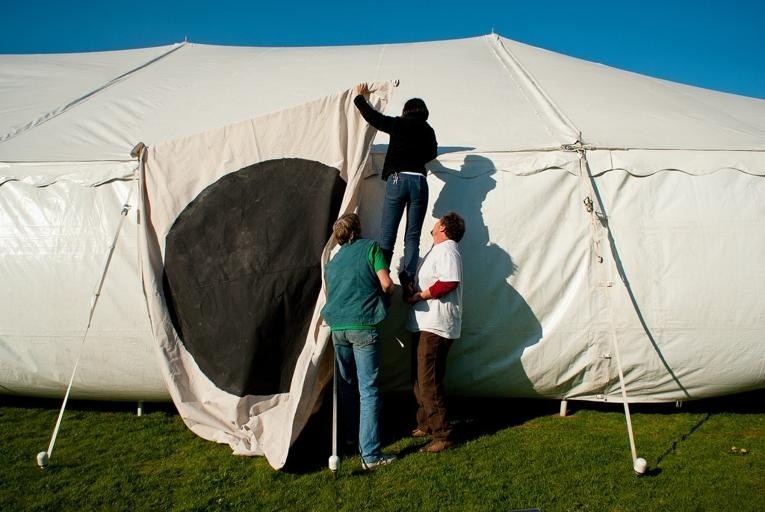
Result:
[361,454,398,470]
[410,428,432,438]
[424,438,449,453]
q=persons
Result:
[319,210,398,472]
[404,209,467,453]
[352,81,440,297]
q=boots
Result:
[399,271,419,303]
